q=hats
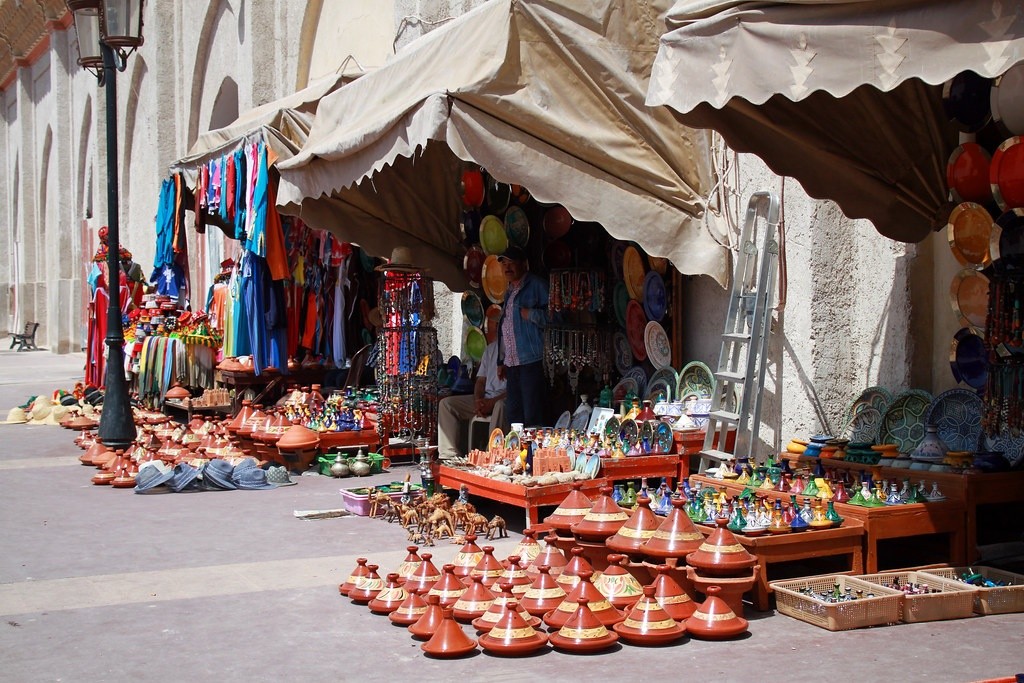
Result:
[133,458,278,494]
[267,466,298,486]
[374,246,432,273]
[84,387,104,406]
[26,394,95,425]
[498,245,528,262]
[0,407,27,424]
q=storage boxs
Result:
[852,571,977,622]
[770,575,904,631]
[340,486,426,517]
[317,453,384,477]
[917,565,1024,616]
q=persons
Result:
[438,314,507,461]
[496,246,550,428]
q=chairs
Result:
[468,415,492,452]
[322,344,372,394]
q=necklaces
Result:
[547,271,609,313]
[543,326,617,394]
[377,278,438,440]
[983,277,1024,354]
[980,364,1024,438]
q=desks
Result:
[615,452,1024,611]
[163,396,235,423]
[433,430,736,539]
[221,369,348,418]
[320,429,381,454]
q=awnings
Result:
[166,71,365,195]
[276,0,729,291]
[645,0,1024,244]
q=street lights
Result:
[65,0,151,448]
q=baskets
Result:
[769,575,904,632]
[852,571,980,623]
[349,453,385,473]
[917,566,1024,615]
[317,453,348,476]
[339,484,423,516]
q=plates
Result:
[842,60,1024,462]
[435,157,719,456]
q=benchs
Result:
[7,322,40,352]
